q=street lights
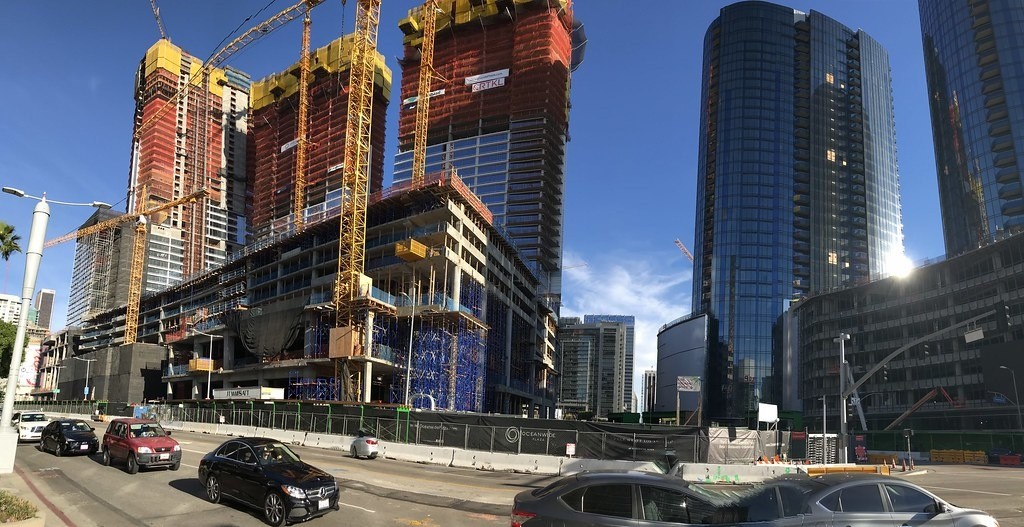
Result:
[1,187,112,474]
[399,288,416,409]
[834,333,851,464]
[817,393,828,464]
[73,356,97,400]
[999,365,1023,429]
[190,328,223,400]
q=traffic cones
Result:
[883,459,887,466]
[891,458,896,469]
[901,459,908,471]
[911,459,915,471]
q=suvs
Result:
[102,418,182,475]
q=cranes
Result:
[44,183,210,347]
[134,1,323,233]
[410,0,438,190]
[333,0,382,327]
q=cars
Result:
[198,436,340,527]
[39,418,100,456]
[508,469,728,526]
[348,436,380,460]
[12,412,51,443]
[695,473,1001,527]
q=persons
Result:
[241,448,255,463]
[117,424,136,438]
[141,423,156,436]
[259,446,282,464]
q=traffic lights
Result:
[882,364,889,384]
[847,400,854,421]
[919,342,930,361]
